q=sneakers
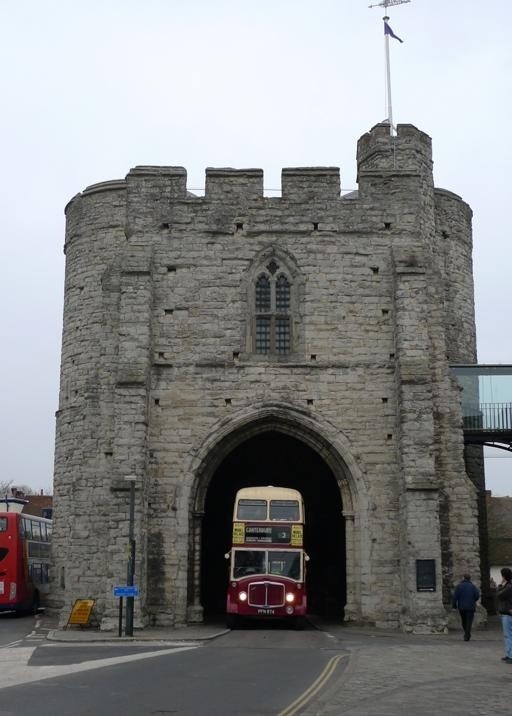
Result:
[501,656,512,664]
[464,638,469,641]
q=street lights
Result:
[123,464,138,636]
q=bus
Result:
[222,483,317,631]
[1,510,55,618]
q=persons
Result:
[492,568,511,664]
[450,573,481,643]
